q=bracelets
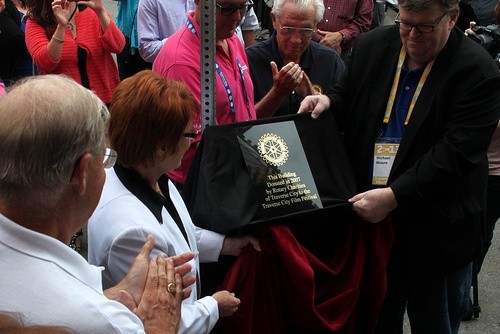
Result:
[51,35,64,43]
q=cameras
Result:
[468,24,500,59]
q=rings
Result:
[166,282,176,293]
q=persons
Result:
[0,0,500,334]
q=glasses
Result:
[177,130,200,149]
[277,17,317,39]
[394,9,450,34]
[216,0,254,16]
[69,147,118,182]
